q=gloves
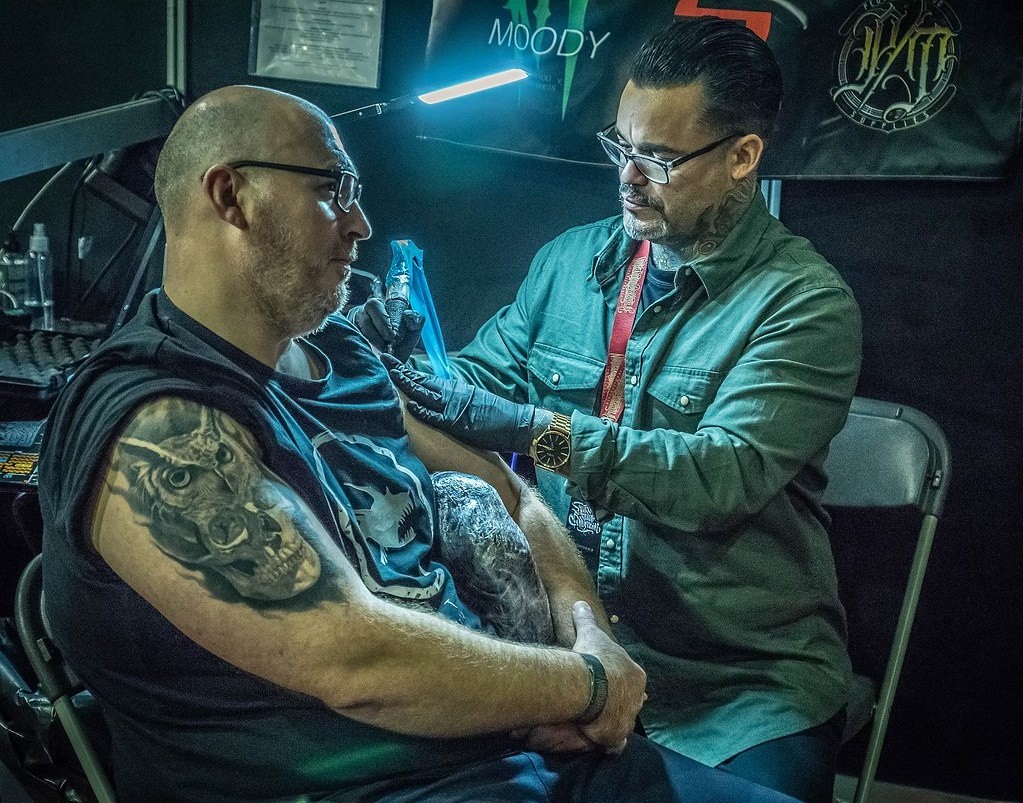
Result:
[379,347,539,458]
[347,297,425,366]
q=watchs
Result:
[534,411,572,472]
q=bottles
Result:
[24,223,55,330]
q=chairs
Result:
[16,546,120,803]
[817,396,956,802]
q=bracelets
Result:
[575,653,608,727]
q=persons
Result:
[38,83,800,802]
[350,16,863,803]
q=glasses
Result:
[593,122,745,188]
[198,160,367,217]
